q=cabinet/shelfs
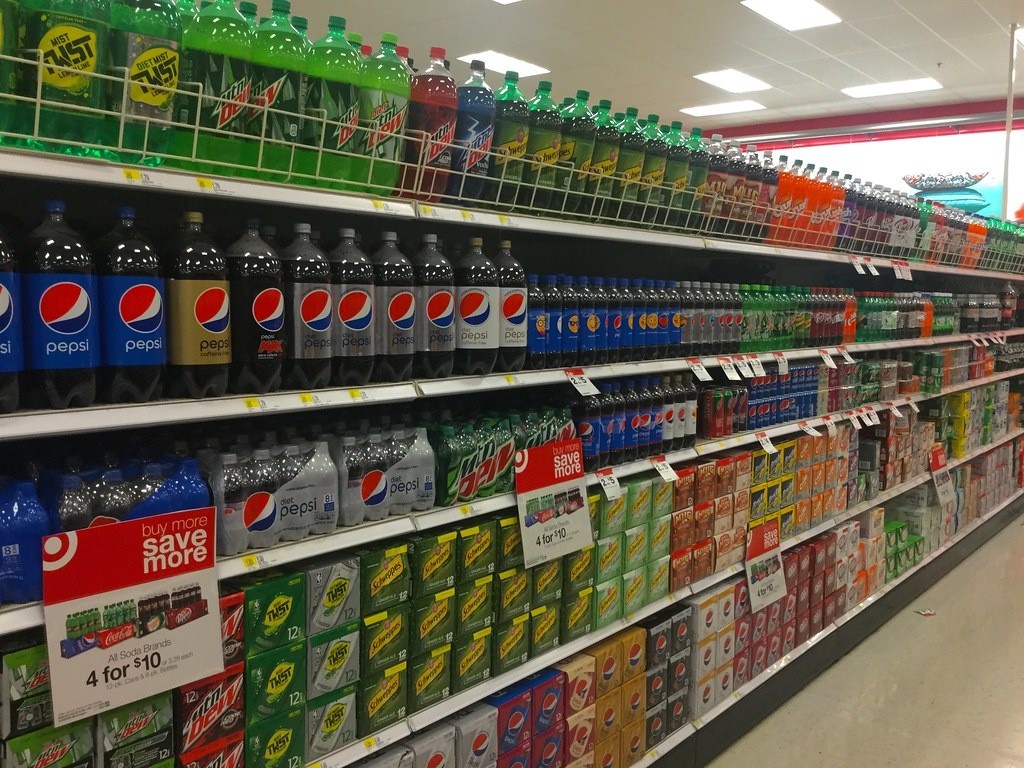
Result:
[0,47,1024,768]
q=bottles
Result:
[0,0,1024,604]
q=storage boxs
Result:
[0,380,1023,767]
